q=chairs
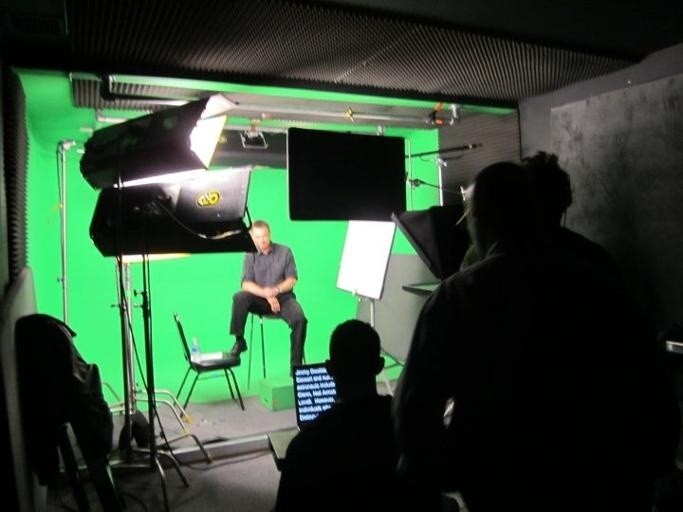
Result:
[173,313,245,418]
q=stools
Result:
[248,313,306,388]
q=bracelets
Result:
[275,284,282,295]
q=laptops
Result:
[292,363,336,432]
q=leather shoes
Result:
[231,341,248,356]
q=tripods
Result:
[102,256,213,512]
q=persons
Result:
[228,219,307,380]
[270,318,399,511]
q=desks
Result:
[267,428,300,472]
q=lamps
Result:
[79,93,260,257]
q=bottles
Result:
[191,338,200,364]
